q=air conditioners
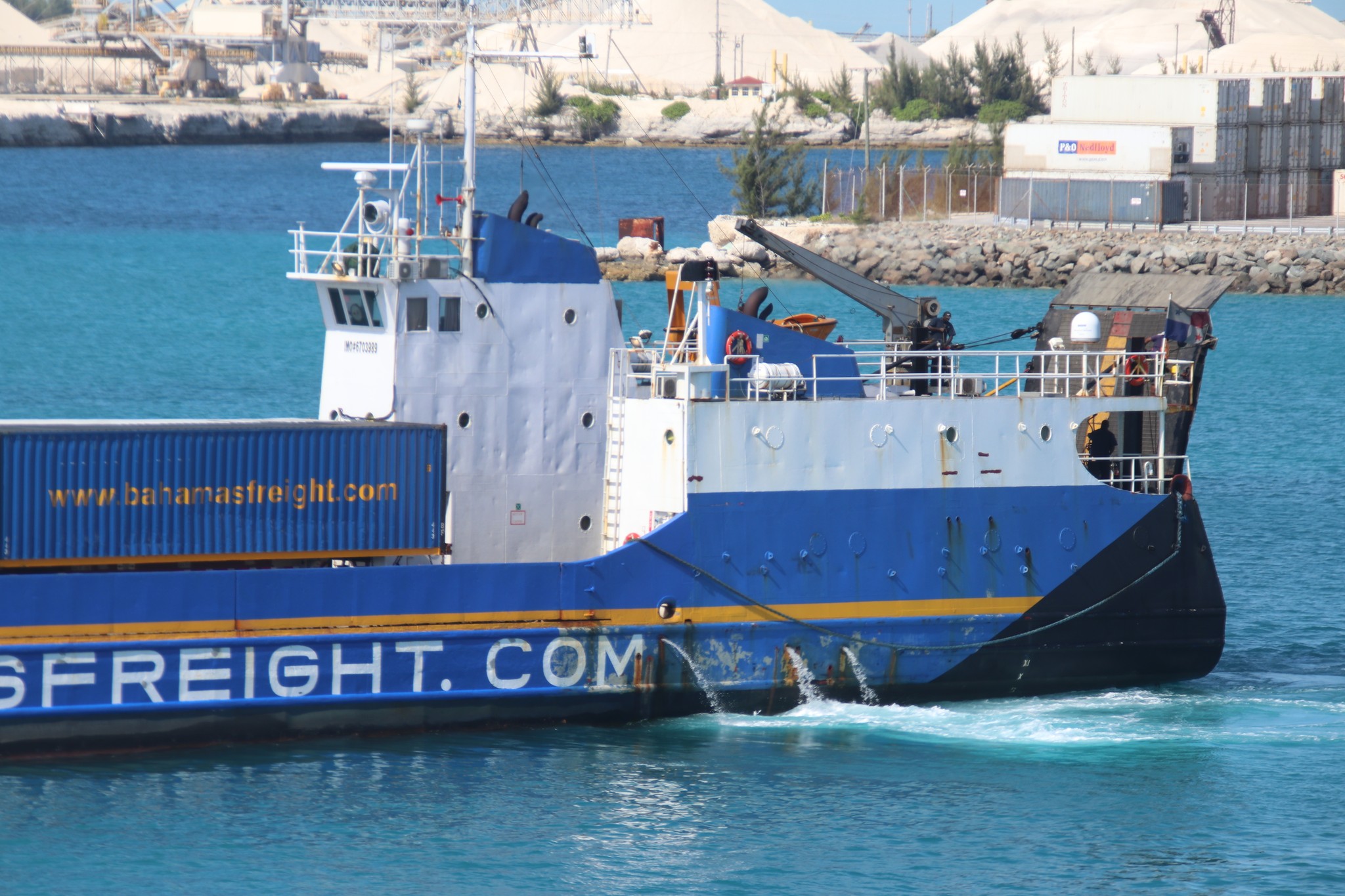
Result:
[390,260,419,280]
[655,375,676,398]
[955,374,982,396]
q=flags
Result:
[1165,301,1204,345]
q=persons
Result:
[344,237,381,279]
[927,311,956,386]
[1145,331,1168,386]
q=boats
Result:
[0,25,1237,761]
[664,313,837,363]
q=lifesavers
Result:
[725,329,752,365]
[1124,355,1148,386]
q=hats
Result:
[944,311,951,319]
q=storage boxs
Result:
[1000,75,1345,223]
[0,418,451,572]
